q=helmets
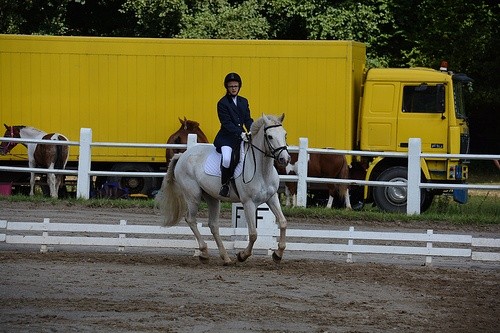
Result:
[224,73,242,87]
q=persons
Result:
[213,72,254,197]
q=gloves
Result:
[240,132,249,144]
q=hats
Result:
[227,81,239,87]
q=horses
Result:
[0,123,70,200]
[275,144,354,211]
[166,116,209,154]
[151,112,292,266]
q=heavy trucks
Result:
[1,32,472,214]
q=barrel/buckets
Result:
[0,182,13,196]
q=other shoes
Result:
[220,186,227,196]
